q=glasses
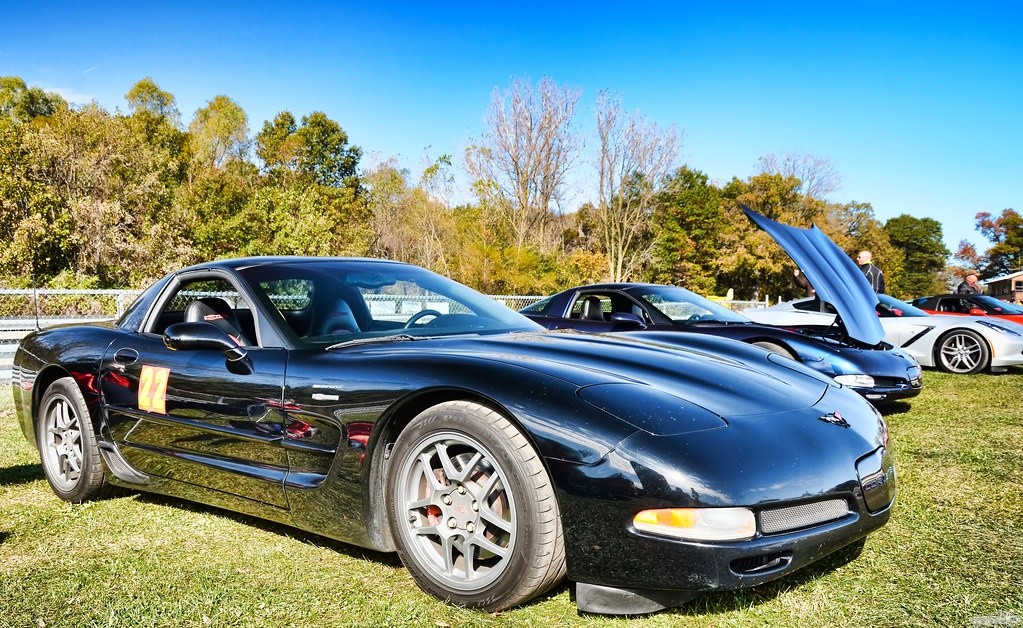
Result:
[858,256,865,259]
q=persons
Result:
[959,270,982,310]
[857,251,885,294]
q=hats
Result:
[964,270,981,276]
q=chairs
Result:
[310,298,361,339]
[940,303,951,311]
[580,296,606,321]
[184,297,252,347]
[632,295,653,325]
[952,303,962,311]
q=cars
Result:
[904,294,1023,327]
[736,294,990,374]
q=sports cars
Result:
[520,202,924,403]
[11,255,896,614]
